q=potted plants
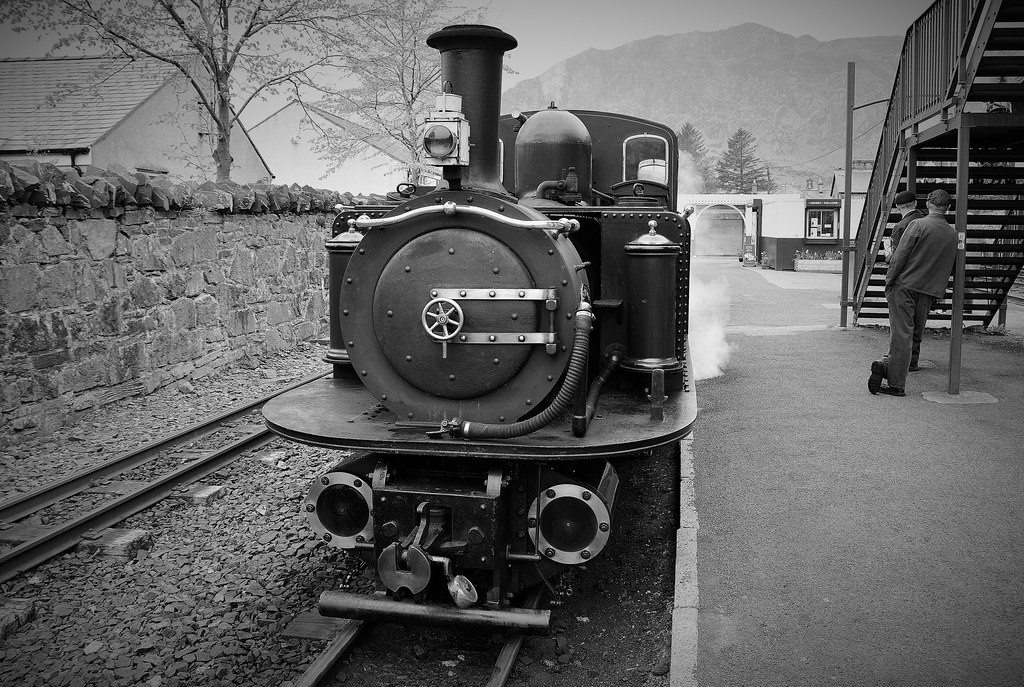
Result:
[793,250,842,274]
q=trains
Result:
[260,22,701,640]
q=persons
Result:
[867,189,959,397]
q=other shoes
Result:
[908,361,918,371]
[878,384,906,396]
[868,360,887,395]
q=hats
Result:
[927,189,951,207]
[894,191,916,204]
